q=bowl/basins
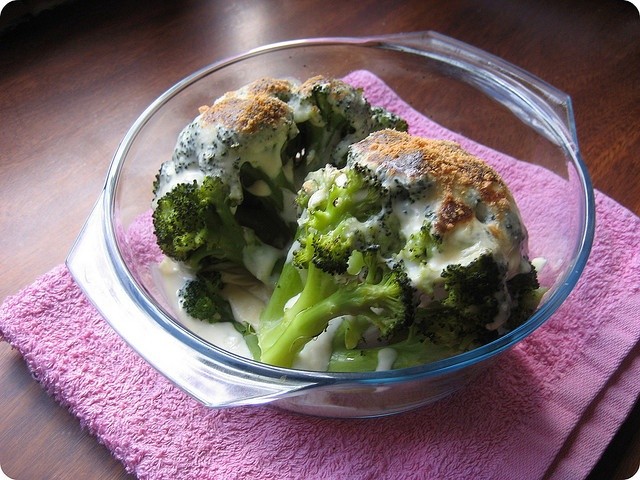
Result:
[60,27,596,419]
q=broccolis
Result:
[260,129,546,370]
[150,76,409,290]
[178,262,261,360]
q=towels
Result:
[0,69,640,480]
